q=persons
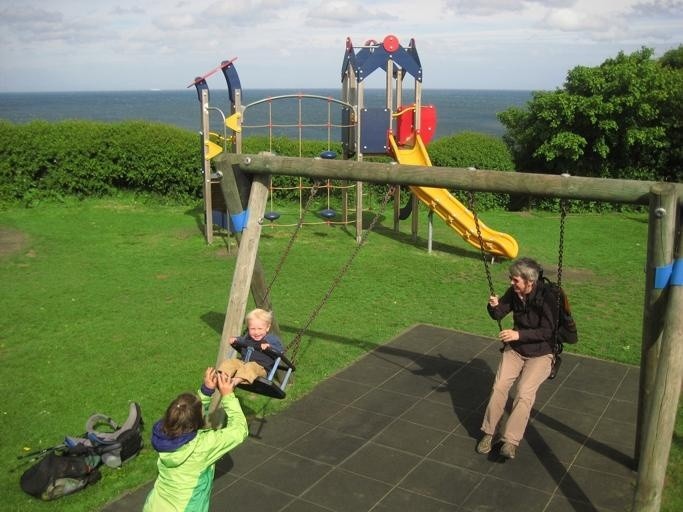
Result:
[207,308,285,393]
[140,365,249,511]
[476,256,562,459]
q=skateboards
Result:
[388,134,521,261]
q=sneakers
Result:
[496,440,517,460]
[476,432,501,454]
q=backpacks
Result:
[537,277,577,344]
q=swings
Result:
[468,190,568,379]
[231,182,394,397]
